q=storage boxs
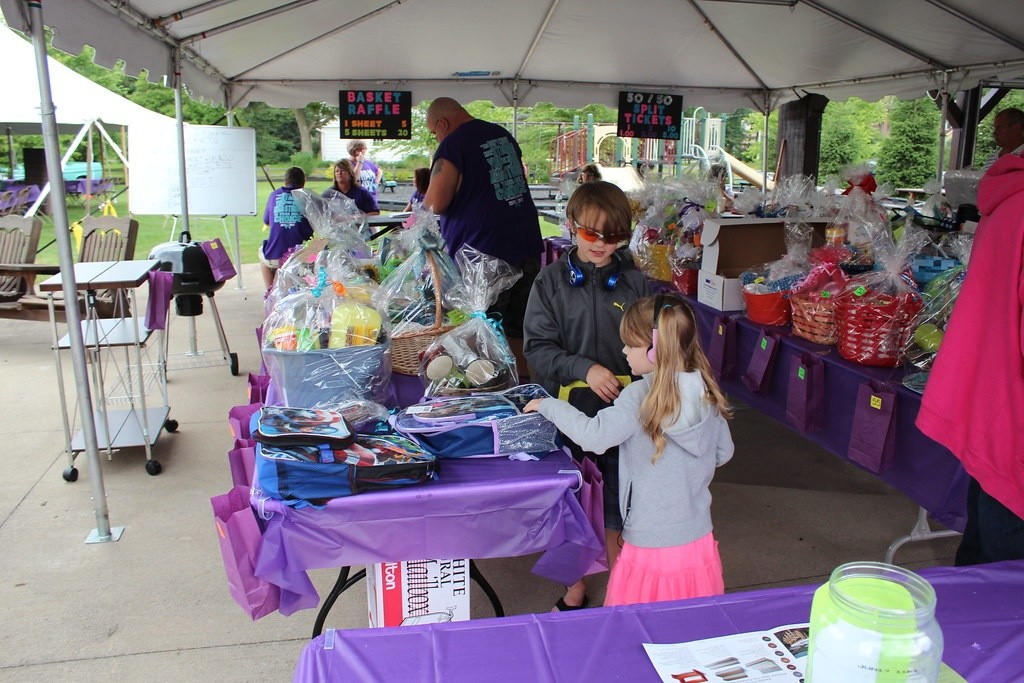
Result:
[698,214,839,310]
[366,558,471,628]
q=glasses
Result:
[991,125,1009,130]
[581,173,594,177]
[573,220,631,246]
[430,120,441,139]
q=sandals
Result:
[552,594,588,611]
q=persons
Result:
[402,167,430,213]
[258,166,313,290]
[982,108,1024,171]
[422,97,546,378]
[915,148,1024,567]
[321,160,380,237]
[522,179,654,613]
[349,141,382,207]
[522,294,734,608]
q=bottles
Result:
[825,227,845,247]
[802,562,943,683]
[555,192,562,213]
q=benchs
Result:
[381,172,397,193]
[0,215,139,363]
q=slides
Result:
[595,163,642,192]
[709,145,775,191]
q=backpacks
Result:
[249,383,564,510]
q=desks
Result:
[358,217,440,239]
[253,372,603,637]
[541,236,970,569]
[0,185,40,214]
[292,560,1024,683]
[65,178,114,196]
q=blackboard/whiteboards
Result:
[127,122,258,216]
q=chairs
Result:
[0,186,52,224]
[62,177,117,211]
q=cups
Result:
[426,353,507,389]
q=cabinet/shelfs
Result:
[39,260,181,481]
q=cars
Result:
[0,158,102,197]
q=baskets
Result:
[741,256,965,368]
[389,248,477,376]
